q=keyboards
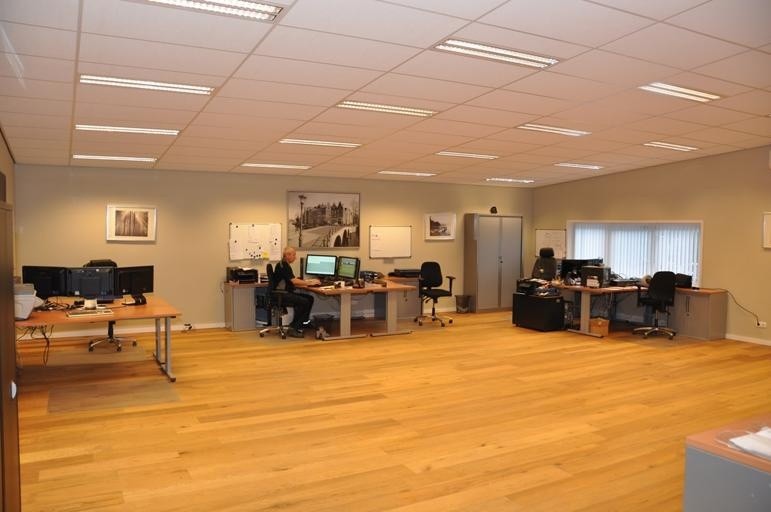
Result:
[307,282,334,287]
[74,299,113,306]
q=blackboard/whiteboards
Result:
[535,229,567,260]
[369,226,412,258]
[229,223,282,261]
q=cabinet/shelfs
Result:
[681,419,771,512]
[463,214,523,313]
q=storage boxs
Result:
[589,317,610,336]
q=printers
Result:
[516,278,540,296]
[13,283,45,321]
[229,268,258,284]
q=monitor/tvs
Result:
[22,265,67,311]
[114,265,154,306]
[66,266,117,318]
[305,254,337,283]
[337,256,360,286]
[560,259,604,286]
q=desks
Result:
[222,278,377,332]
[376,274,424,324]
[365,279,417,337]
[307,282,373,341]
[564,282,729,343]
[14,292,181,383]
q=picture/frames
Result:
[104,204,157,243]
[285,189,362,252]
[426,210,457,241]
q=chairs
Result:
[529,247,557,279]
[630,271,677,340]
[259,263,304,338]
[414,261,455,327]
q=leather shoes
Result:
[302,320,321,330]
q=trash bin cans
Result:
[312,314,333,340]
[455,295,472,313]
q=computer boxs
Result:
[581,265,611,288]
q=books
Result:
[728,428,770,460]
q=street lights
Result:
[298,194,307,247]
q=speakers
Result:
[300,257,304,280]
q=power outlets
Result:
[755,321,767,328]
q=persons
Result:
[266,246,320,339]
